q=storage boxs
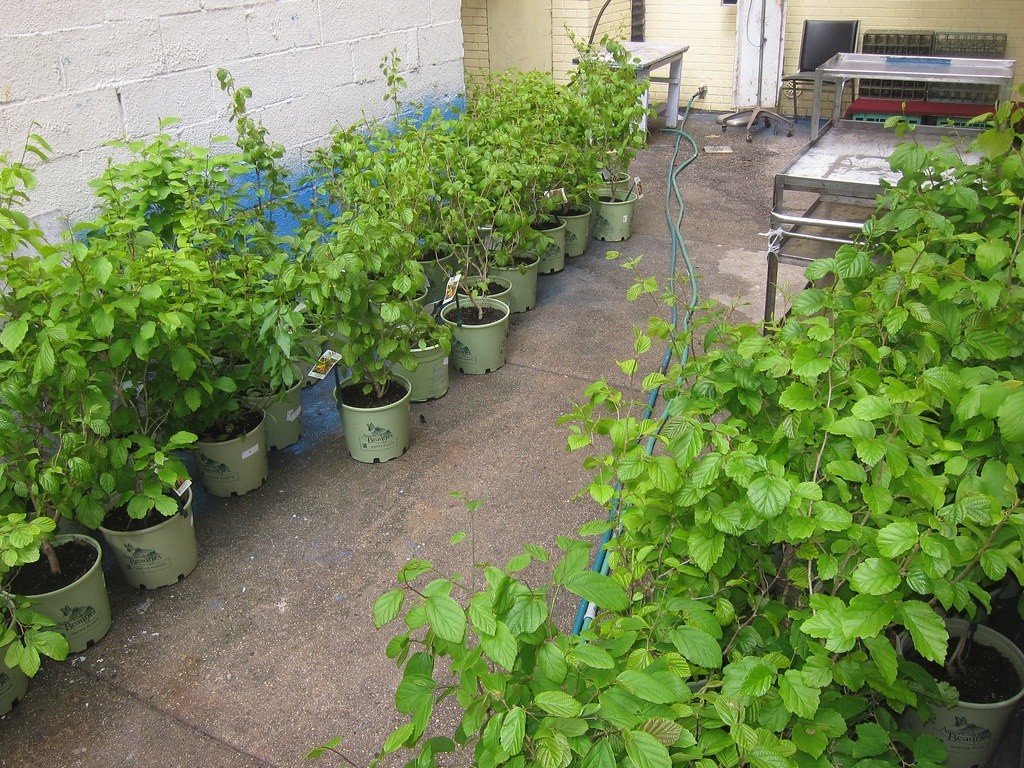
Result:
[852,29,1008,129]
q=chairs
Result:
[773,19,862,138]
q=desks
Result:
[571,42,690,148]
[762,119,1012,337]
[811,53,1017,136]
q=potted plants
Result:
[0,12,659,715]
[787,336,1023,768]
